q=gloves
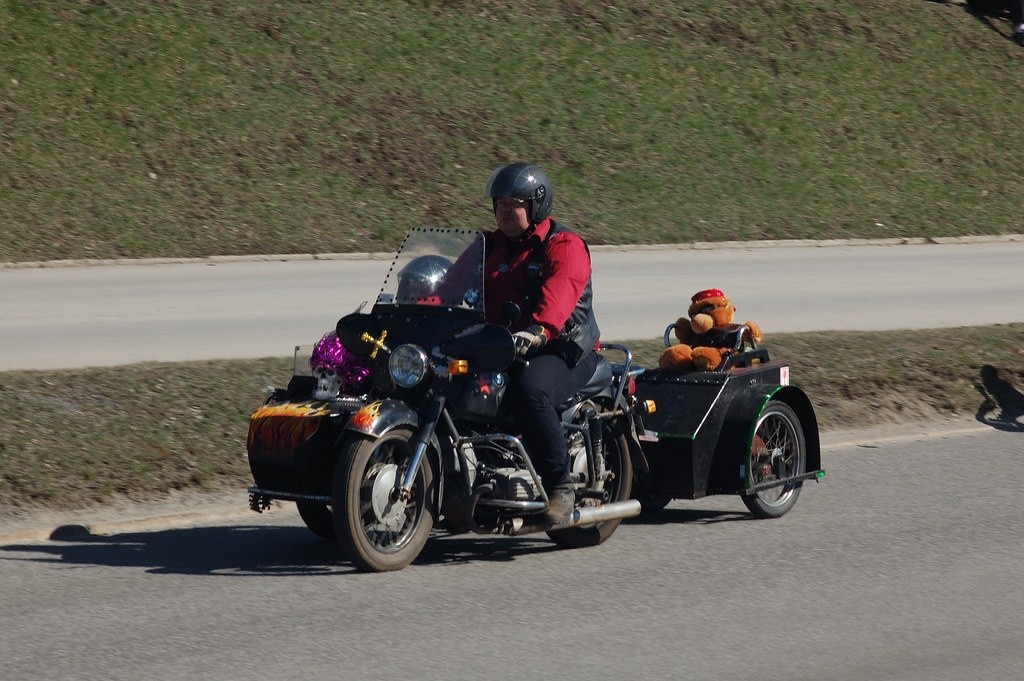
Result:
[512,323,548,357]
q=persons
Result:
[412,162,601,525]
[395,254,454,305]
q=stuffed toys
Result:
[659,288,762,376]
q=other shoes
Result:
[542,476,576,524]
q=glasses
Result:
[493,198,530,210]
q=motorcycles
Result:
[246,227,825,573]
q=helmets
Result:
[484,161,553,222]
[396,255,453,302]
